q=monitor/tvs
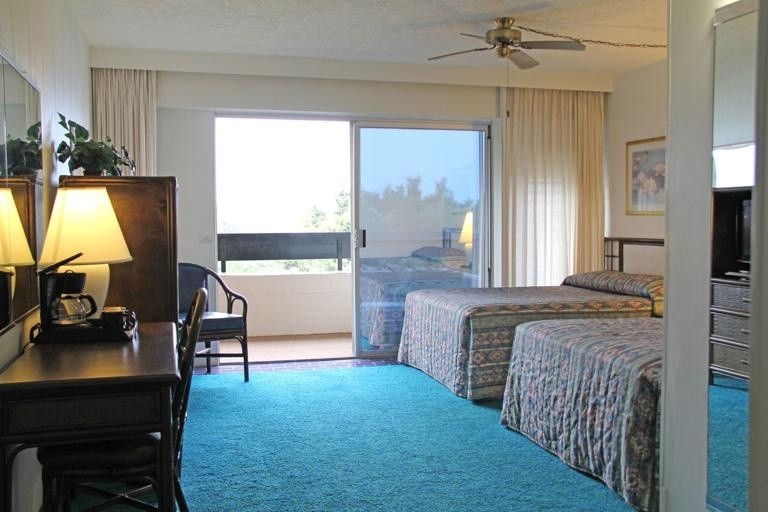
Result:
[734,195,751,264]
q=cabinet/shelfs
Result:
[709,187,750,389]
[60,177,177,325]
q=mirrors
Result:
[0,51,52,334]
[706,1,756,509]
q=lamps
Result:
[459,212,474,264]
[38,187,133,320]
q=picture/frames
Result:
[626,136,665,216]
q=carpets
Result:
[37,365,645,511]
[708,374,749,512]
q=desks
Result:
[0,322,180,511]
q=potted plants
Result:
[56,112,136,180]
[0,121,42,177]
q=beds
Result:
[359,229,471,351]
[509,316,663,480]
[402,283,653,400]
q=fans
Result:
[429,17,584,70]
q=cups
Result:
[101,306,136,331]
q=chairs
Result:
[178,263,250,382]
[37,288,208,510]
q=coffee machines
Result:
[30,252,138,342]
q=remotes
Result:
[725,271,751,279]
